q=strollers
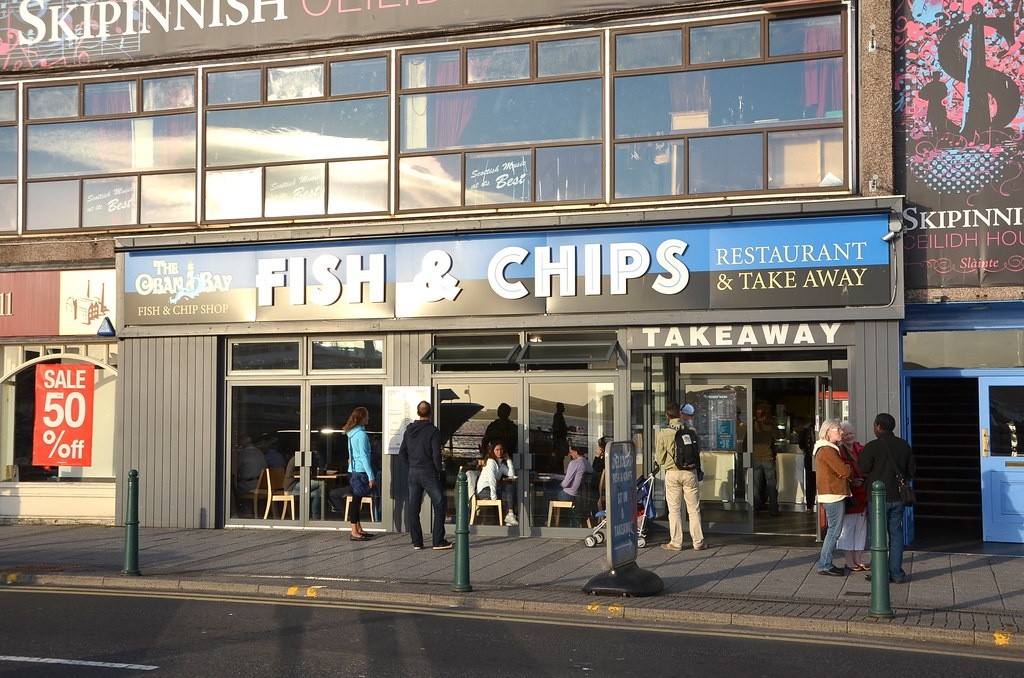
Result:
[584,461,660,549]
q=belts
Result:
[245,476,259,481]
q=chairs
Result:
[236,467,295,520]
[294,472,349,521]
[466,470,503,526]
[548,469,606,528]
[344,471,381,522]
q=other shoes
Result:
[754,508,757,515]
[312,512,321,520]
[769,509,784,517]
[413,543,423,550]
[238,511,252,518]
[818,565,845,576]
[350,534,371,541]
[660,543,682,551]
[433,540,455,550]
[889,568,905,582]
[806,509,813,514]
[358,532,373,538]
[504,514,519,526]
[230,511,240,518]
[330,505,341,512]
[694,543,710,551]
[505,517,516,526]
[853,560,871,571]
[845,563,863,572]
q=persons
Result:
[839,421,870,571]
[813,419,852,576]
[330,484,353,518]
[232,432,285,516]
[742,404,785,514]
[544,442,592,526]
[283,440,335,519]
[857,413,914,583]
[592,436,615,474]
[477,442,519,526]
[344,407,375,539]
[799,409,815,513]
[399,401,454,549]
[657,402,708,550]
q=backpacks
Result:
[664,425,700,470]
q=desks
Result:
[502,476,565,527]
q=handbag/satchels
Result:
[899,485,916,507]
[351,471,372,496]
[844,497,857,511]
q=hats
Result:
[680,404,696,415]
